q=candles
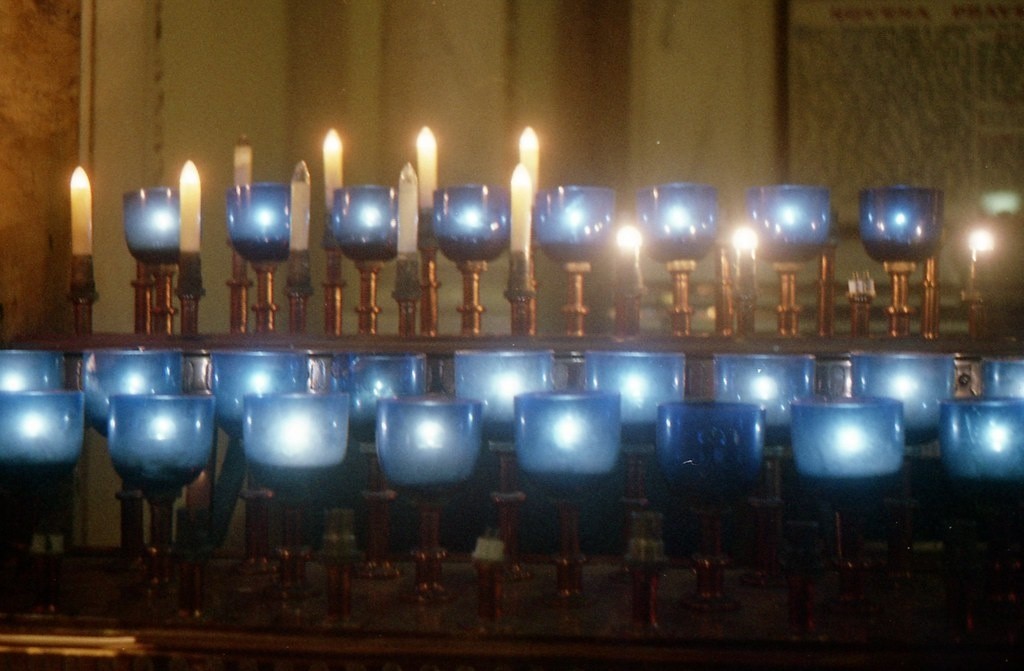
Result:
[180,160,200,253]
[290,161,310,250]
[233,136,251,185]
[70,167,93,255]
[519,126,540,196]
[510,165,533,260]
[398,163,419,251]
[323,129,344,210]
[417,126,438,208]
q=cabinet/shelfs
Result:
[0,326,1023,671]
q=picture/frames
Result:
[777,1,1024,237]
